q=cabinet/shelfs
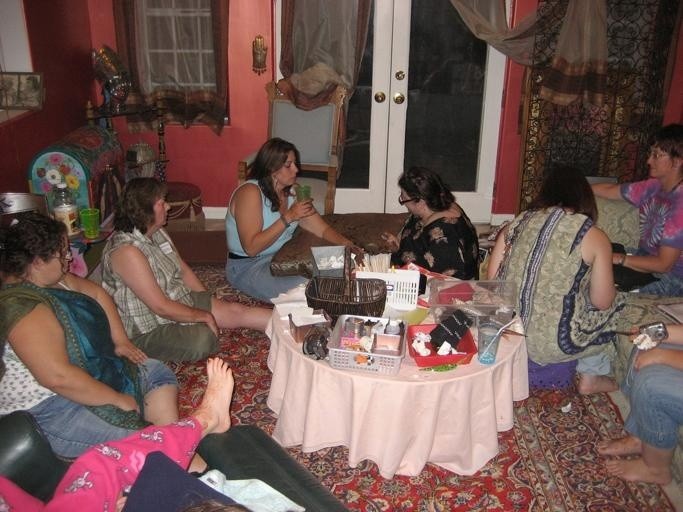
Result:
[82,95,171,222]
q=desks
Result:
[265,264,527,481]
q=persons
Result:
[598,321,682,485]
[383,165,480,296]
[591,122,683,297]
[2,214,182,460]
[22,76,40,106]
[1,76,17,106]
[0,354,237,512]
[488,164,619,395]
[183,496,248,512]
[101,176,274,361]
[222,136,363,307]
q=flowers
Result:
[35,152,83,200]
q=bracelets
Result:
[621,254,626,265]
[281,215,291,228]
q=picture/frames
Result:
[0,69,46,112]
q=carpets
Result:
[107,258,677,512]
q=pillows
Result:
[119,452,248,512]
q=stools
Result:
[157,181,204,222]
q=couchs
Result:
[587,194,683,495]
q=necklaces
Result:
[421,210,435,226]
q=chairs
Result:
[238,76,350,221]
[0,407,348,511]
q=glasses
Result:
[646,150,669,160]
[399,196,412,206]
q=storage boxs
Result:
[424,275,517,328]
[404,321,476,366]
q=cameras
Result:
[302,327,329,361]
[639,322,669,342]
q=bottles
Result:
[54,182,80,241]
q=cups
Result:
[419,274,426,294]
[477,323,501,362]
[79,207,99,238]
[295,185,310,203]
